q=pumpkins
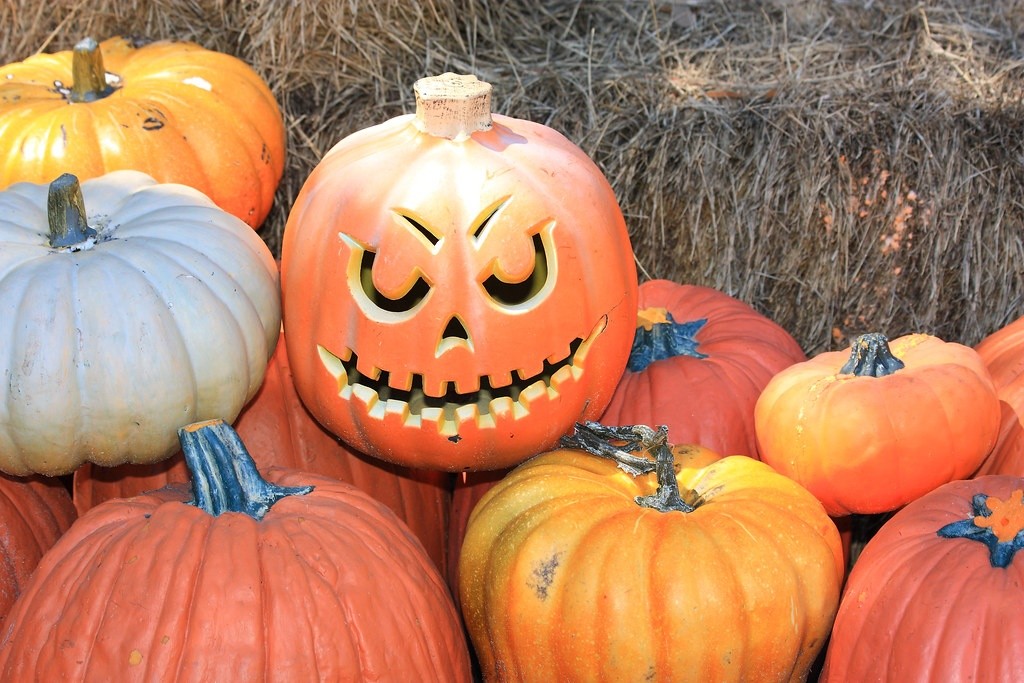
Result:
[0,36,1023,683]
